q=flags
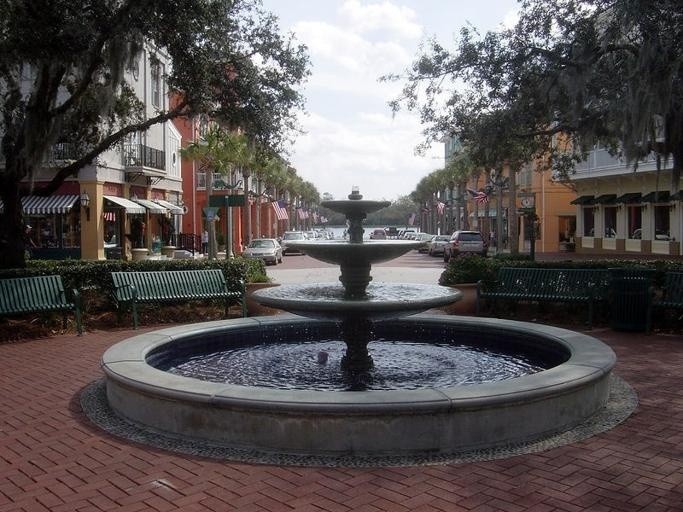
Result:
[435,200,447,216]
[466,189,489,207]
[271,199,288,221]
[407,212,416,227]
[295,206,328,225]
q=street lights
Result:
[485,174,510,254]
[247,186,270,239]
[446,192,469,231]
[209,179,243,265]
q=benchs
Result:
[0,269,247,337]
[475,266,682,336]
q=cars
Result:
[241,227,335,267]
[589,227,616,238]
[630,227,669,240]
[367,223,489,264]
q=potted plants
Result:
[245,272,281,316]
[438,255,482,316]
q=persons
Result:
[24,223,37,259]
[564,227,571,244]
[161,219,171,246]
[129,218,137,248]
[134,217,146,248]
[488,228,495,247]
[201,228,207,257]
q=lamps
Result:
[79,188,90,221]
